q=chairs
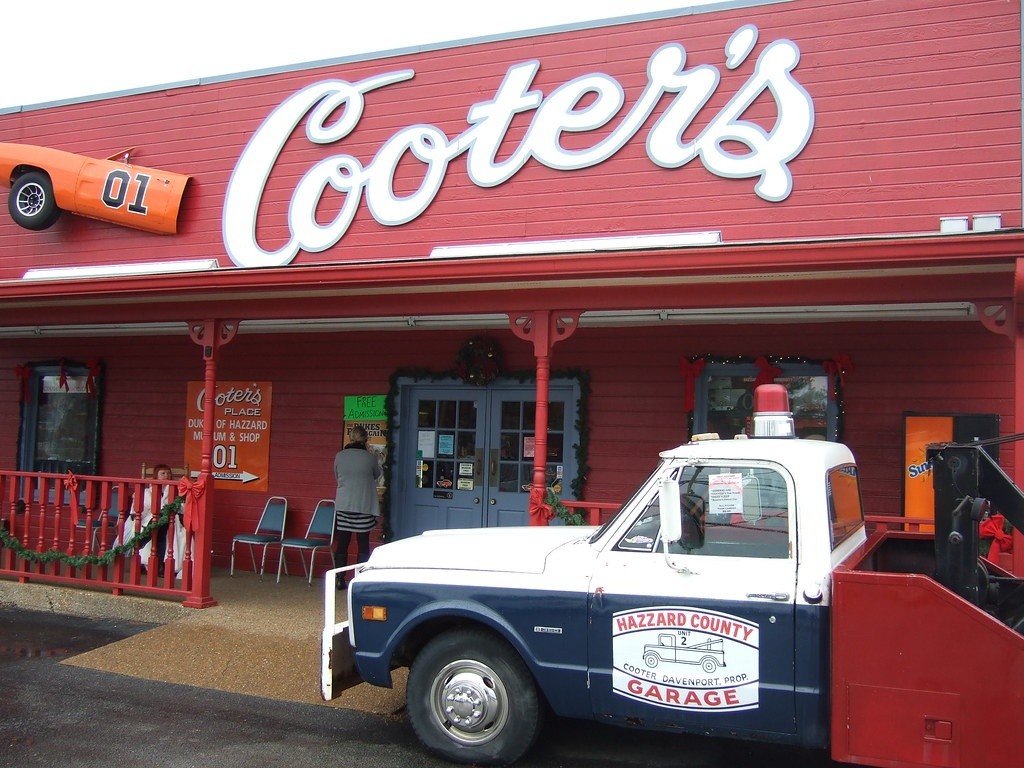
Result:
[75,485,120,553]
[230,496,289,583]
[276,497,338,585]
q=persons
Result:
[333,426,381,590]
[112,464,195,578]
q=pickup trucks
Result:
[321,383,1024,768]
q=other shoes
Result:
[158,564,166,578]
[337,580,347,590]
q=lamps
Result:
[428,231,723,258]
[22,257,220,279]
